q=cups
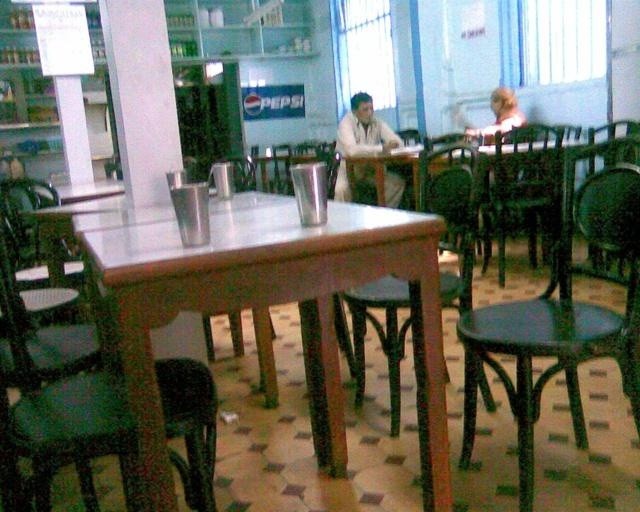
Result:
[279,36,312,53]
[167,161,233,248]
[291,160,328,225]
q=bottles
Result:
[266,145,272,157]
[86,7,107,58]
[0,6,41,64]
[166,9,197,58]
[0,80,17,124]
[263,3,284,25]
[199,5,224,28]
[1,154,24,182]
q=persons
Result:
[330,94,409,209]
[460,87,527,188]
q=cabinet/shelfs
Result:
[0,1,107,130]
[163,0,322,65]
